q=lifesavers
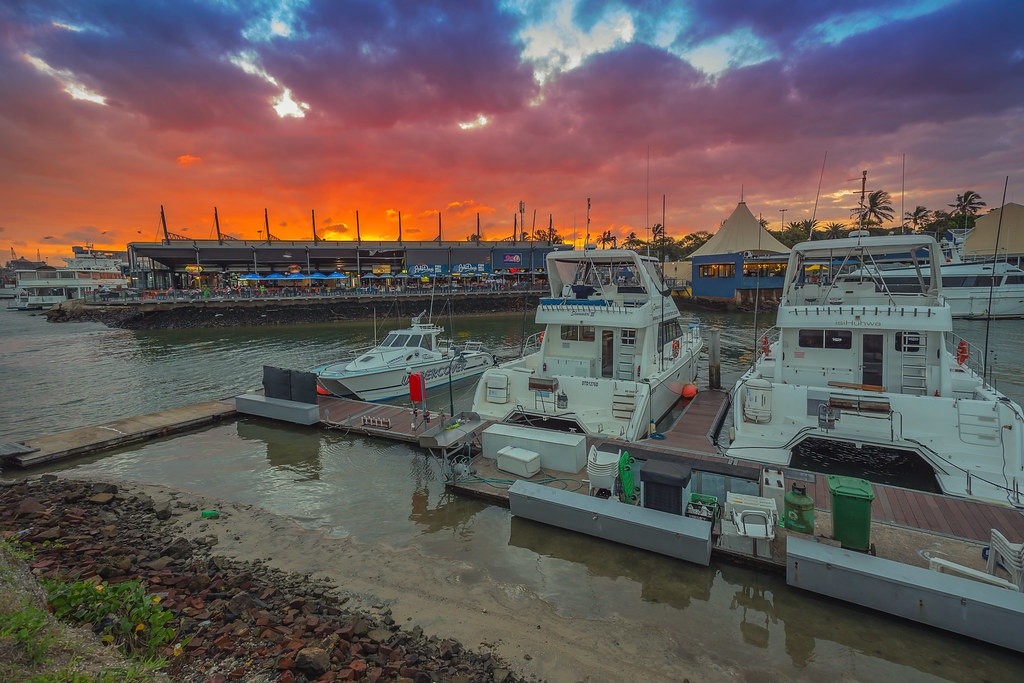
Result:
[672,340,679,358]
[957,340,967,365]
[540,332,544,342]
[762,335,770,356]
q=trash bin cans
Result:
[825,473,876,557]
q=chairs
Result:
[987,528,1024,594]
[729,505,778,541]
[587,445,622,499]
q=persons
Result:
[92,281,551,304]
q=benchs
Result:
[824,392,893,440]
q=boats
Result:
[726,171,1023,508]
[470,245,702,441]
[303,270,494,402]
[10,247,129,310]
[842,263,1023,321]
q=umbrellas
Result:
[239,269,549,288]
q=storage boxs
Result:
[497,446,541,479]
